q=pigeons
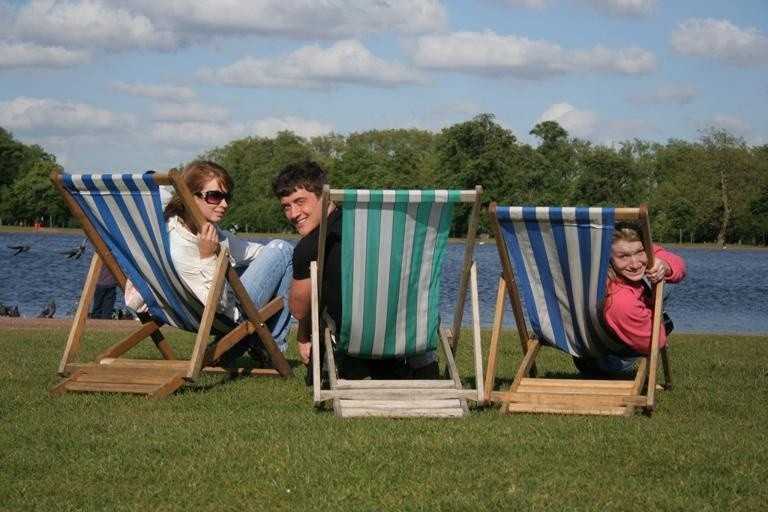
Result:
[0,303,12,315]
[37,299,57,321]
[7,305,20,317]
[59,237,90,262]
[9,238,44,258]
[67,300,133,320]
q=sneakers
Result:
[253,347,272,378]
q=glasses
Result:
[193,190,232,205]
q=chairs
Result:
[483,201,674,418]
[48,169,295,399]
[305,184,485,418]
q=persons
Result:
[126,163,294,356]
[569,221,688,374]
[272,161,343,364]
[94,249,118,317]
